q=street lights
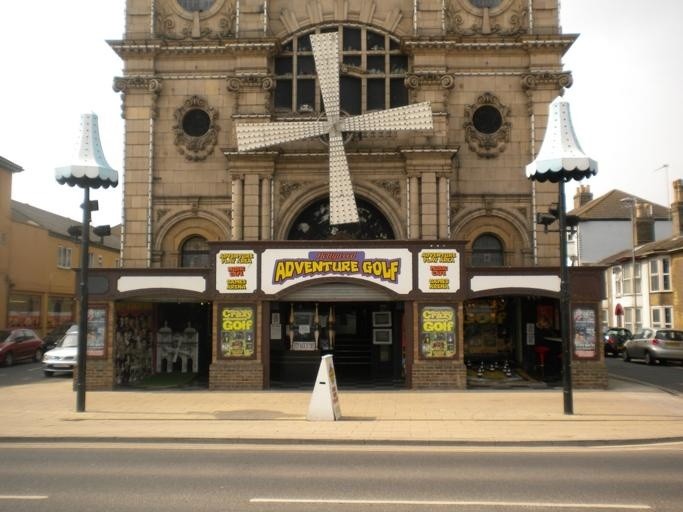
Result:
[524,96,598,412]
[53,109,118,416]
[620,196,637,333]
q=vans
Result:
[575,320,608,336]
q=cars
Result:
[621,326,682,364]
[42,332,78,376]
[603,327,633,357]
[0,327,45,366]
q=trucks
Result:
[41,321,105,353]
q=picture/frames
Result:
[372,312,392,327]
[372,329,393,345]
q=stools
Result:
[535,345,550,379]
